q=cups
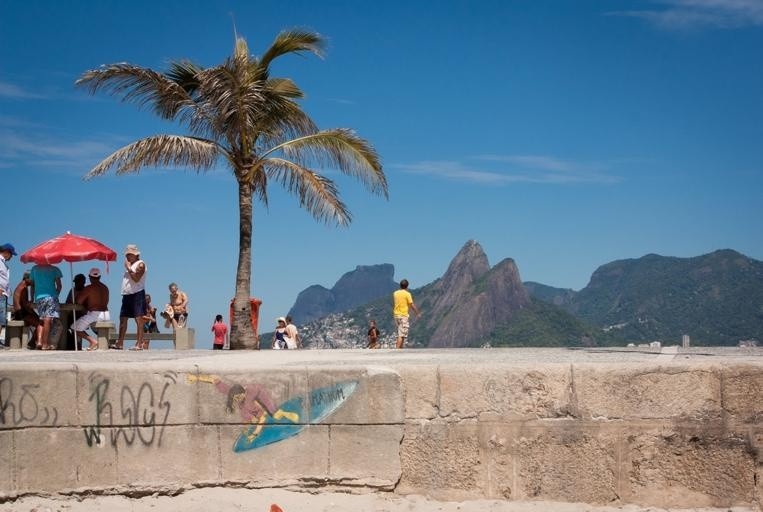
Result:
[7,311,12,321]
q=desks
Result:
[30,302,83,349]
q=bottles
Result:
[178,312,184,327]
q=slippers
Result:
[109,344,124,349]
[87,343,98,351]
[129,346,142,351]
[36,343,56,350]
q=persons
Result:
[110,243,148,350]
[65,272,88,350]
[28,263,64,351]
[11,271,44,349]
[283,316,302,349]
[271,317,287,349]
[141,294,158,350]
[391,279,420,349]
[69,268,110,352]
[366,320,379,350]
[188,373,301,446]
[211,314,229,350]
[0,242,19,350]
[164,282,189,332]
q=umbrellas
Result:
[20,229,120,351]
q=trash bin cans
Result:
[229,297,262,349]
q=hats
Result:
[0,243,18,257]
[276,317,288,327]
[88,268,101,278]
[73,273,86,284]
[122,245,141,257]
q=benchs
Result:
[6,320,24,349]
[108,328,194,350]
[90,320,114,351]
